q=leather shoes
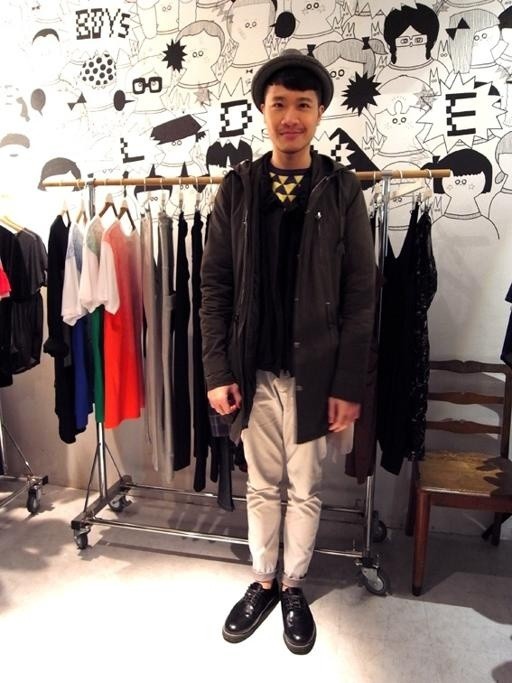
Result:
[222,578,279,644]
[281,587,316,655]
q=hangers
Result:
[1,170,439,251]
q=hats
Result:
[251,49,334,113]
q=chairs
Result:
[405,360,512,597]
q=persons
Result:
[199,48,376,653]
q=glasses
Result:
[133,77,162,94]
